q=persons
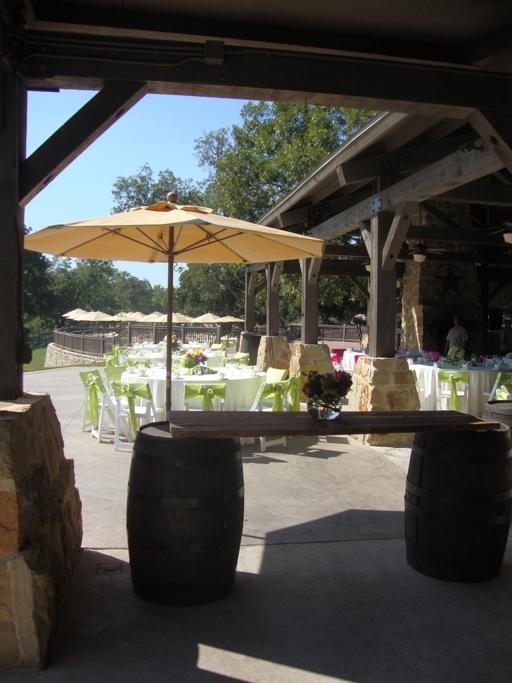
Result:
[443,316,468,355]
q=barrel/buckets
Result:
[402,419,512,583]
[125,421,246,606]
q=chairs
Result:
[409,369,511,416]
[77,336,300,453]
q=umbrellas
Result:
[23,191,324,424]
[62,307,244,345]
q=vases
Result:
[307,393,343,422]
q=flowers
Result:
[300,367,353,413]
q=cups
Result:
[135,370,145,377]
[497,360,504,369]
[126,367,135,374]
